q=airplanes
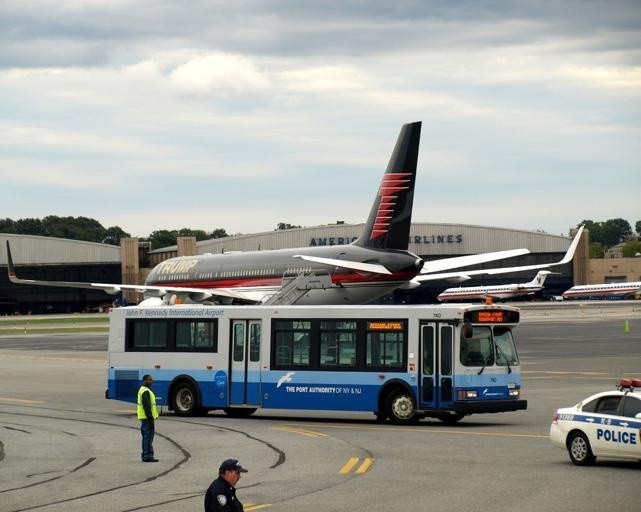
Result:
[5,121,588,307]
[437,269,563,303]
[560,280,641,300]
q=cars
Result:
[549,378,641,465]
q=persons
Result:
[137,375,159,462]
[205,459,248,512]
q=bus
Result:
[104,300,529,426]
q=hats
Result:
[220,459,248,472]
